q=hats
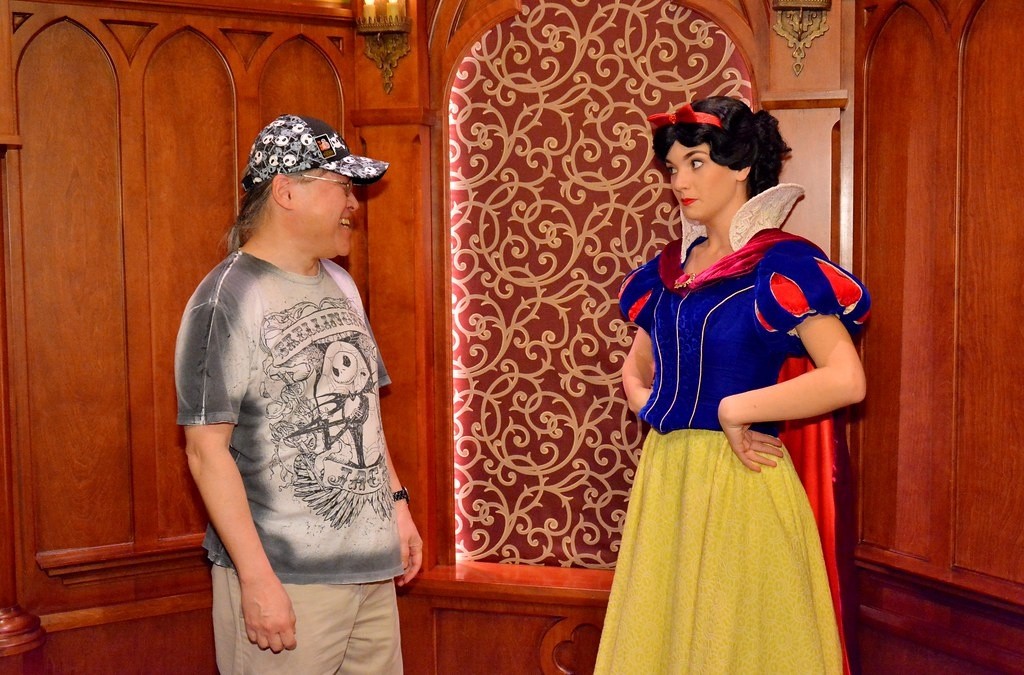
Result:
[241,113,390,192]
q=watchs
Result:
[392,486,410,504]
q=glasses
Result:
[295,168,356,194]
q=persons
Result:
[594,95,872,674]
[174,114,422,675]
